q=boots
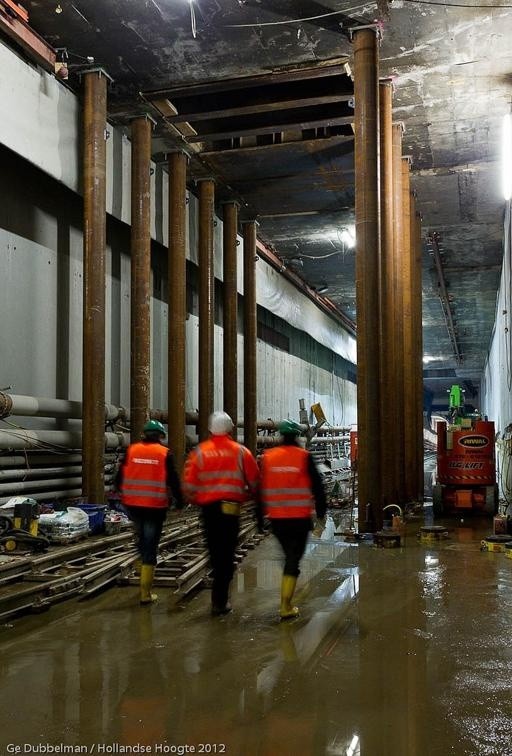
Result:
[278,575,300,618]
[139,562,159,604]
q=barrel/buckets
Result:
[78,503,106,535]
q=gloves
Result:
[312,520,326,536]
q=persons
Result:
[180,411,262,616]
[254,420,328,618]
[114,419,185,604]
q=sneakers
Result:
[209,601,230,616]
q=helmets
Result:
[142,420,166,435]
[277,420,301,435]
[209,411,234,435]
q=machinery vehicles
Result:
[432,410,499,519]
